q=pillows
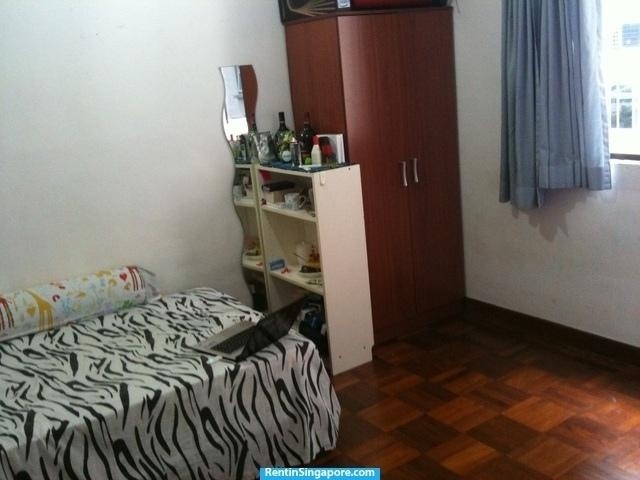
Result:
[2,264,160,340]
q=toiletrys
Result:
[289,134,302,166]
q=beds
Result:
[0,283,341,479]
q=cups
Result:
[283,194,307,209]
[308,189,314,208]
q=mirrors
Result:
[220,62,266,310]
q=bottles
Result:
[252,110,334,168]
[229,125,255,164]
[297,242,310,262]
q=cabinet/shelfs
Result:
[285,5,468,343]
[233,164,264,274]
[253,163,377,375]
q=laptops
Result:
[194,294,308,362]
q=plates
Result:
[299,268,320,277]
